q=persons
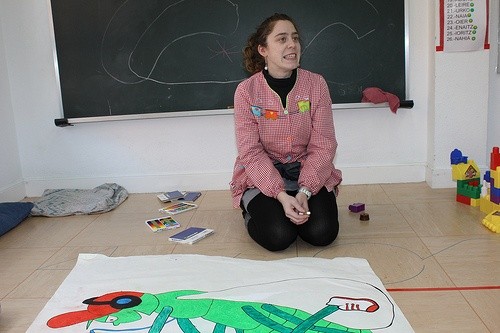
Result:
[230,14,342,252]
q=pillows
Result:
[0,202,33,238]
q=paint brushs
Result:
[298,211,311,214]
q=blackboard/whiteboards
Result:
[47,0,411,127]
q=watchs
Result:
[298,188,312,199]
